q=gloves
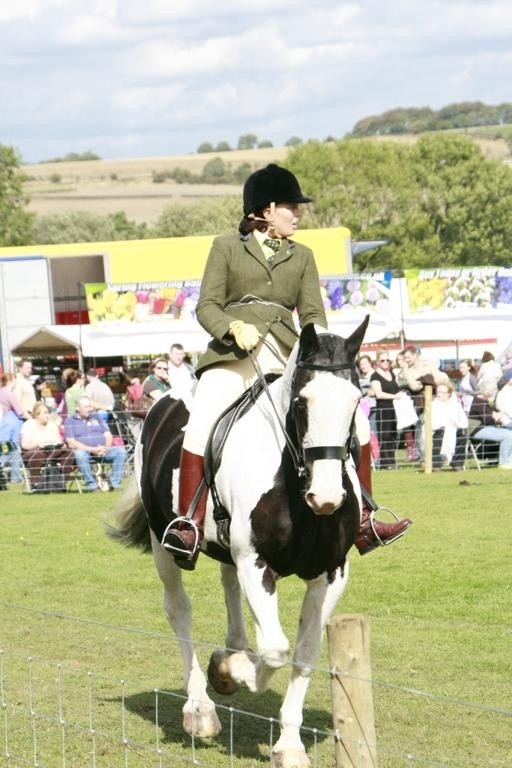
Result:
[228,320,264,352]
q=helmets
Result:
[244,164,312,217]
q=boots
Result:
[163,446,208,570]
[349,441,413,555]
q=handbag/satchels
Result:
[393,391,419,430]
[39,464,66,493]
[132,397,153,418]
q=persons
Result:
[0,343,197,495]
[355,344,512,472]
[163,164,412,573]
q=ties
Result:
[264,240,280,263]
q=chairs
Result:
[369,398,512,470]
[0,394,151,493]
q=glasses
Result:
[380,359,389,364]
[157,367,168,370]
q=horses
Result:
[103,312,372,768]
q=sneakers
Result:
[420,462,425,468]
[441,463,452,470]
[81,474,113,493]
[406,445,416,461]
